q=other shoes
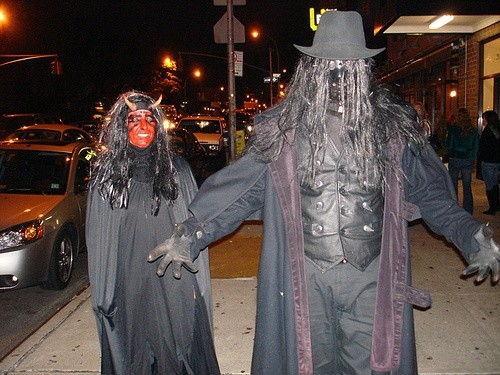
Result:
[483,209,495,215]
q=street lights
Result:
[251,28,280,84]
[159,51,273,108]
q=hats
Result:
[294,11,386,59]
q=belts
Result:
[340,259,348,263]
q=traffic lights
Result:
[51,60,62,76]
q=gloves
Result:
[461,223,500,282]
[148,224,198,278]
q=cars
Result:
[159,101,259,166]
[0,113,102,291]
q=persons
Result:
[412,101,433,151]
[86,90,219,375]
[477,110,500,215]
[443,107,480,216]
[148,11,500,375]
